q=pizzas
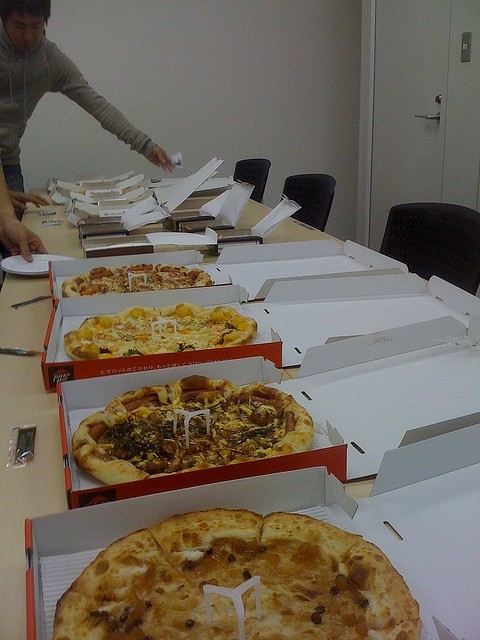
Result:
[63,264,212,296]
[72,374,314,486]
[54,507,419,639]
[65,303,257,360]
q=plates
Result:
[1,254,74,276]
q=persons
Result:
[1,160,49,262]
[0,0,175,221]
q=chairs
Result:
[282,174,336,233]
[233,158,270,202]
[379,203,480,293]
